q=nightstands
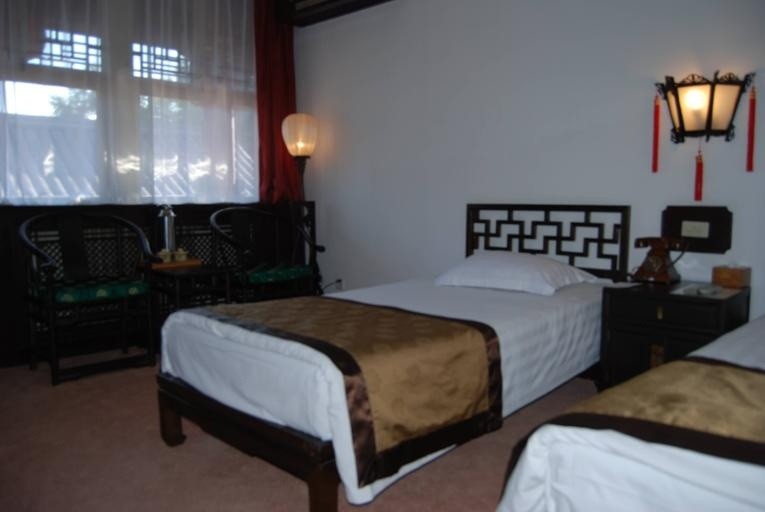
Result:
[600,283,750,389]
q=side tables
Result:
[149,263,232,349]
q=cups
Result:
[158,248,189,263]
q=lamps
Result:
[281,114,319,203]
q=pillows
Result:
[432,250,597,295]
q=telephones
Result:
[632,237,685,283]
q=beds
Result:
[493,315,764,510]
[155,205,632,510]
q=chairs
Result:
[18,214,155,386]
[210,207,324,301]
